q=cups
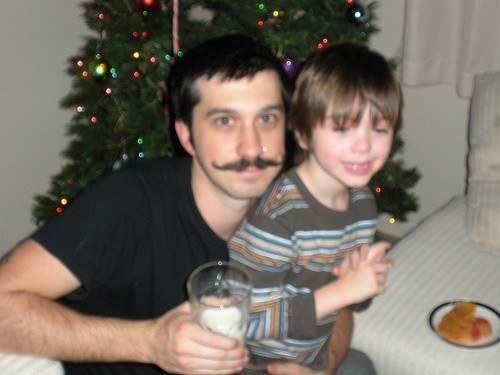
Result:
[187,261,254,346]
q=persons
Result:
[219,41,404,375]
[0,35,377,375]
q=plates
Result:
[429,302,500,351]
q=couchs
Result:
[347,195,499,374]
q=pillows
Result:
[467,71,500,257]
